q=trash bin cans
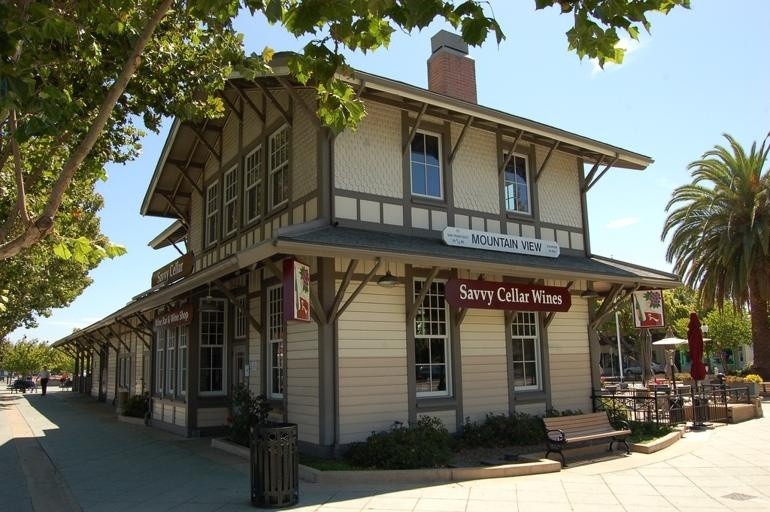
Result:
[250,422,301,509]
[117,392,128,414]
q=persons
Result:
[704,363,709,374]
[35,366,50,396]
[30,375,38,393]
[10,377,18,394]
[59,375,70,391]
[687,310,707,399]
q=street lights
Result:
[701,323,713,376]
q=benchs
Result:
[533,410,634,468]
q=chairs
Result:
[603,381,690,428]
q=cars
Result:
[15,370,88,390]
[624,359,667,374]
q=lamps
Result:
[580,280,600,298]
[376,260,400,285]
[196,285,224,312]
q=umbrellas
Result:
[652,337,687,395]
[681,337,712,344]
